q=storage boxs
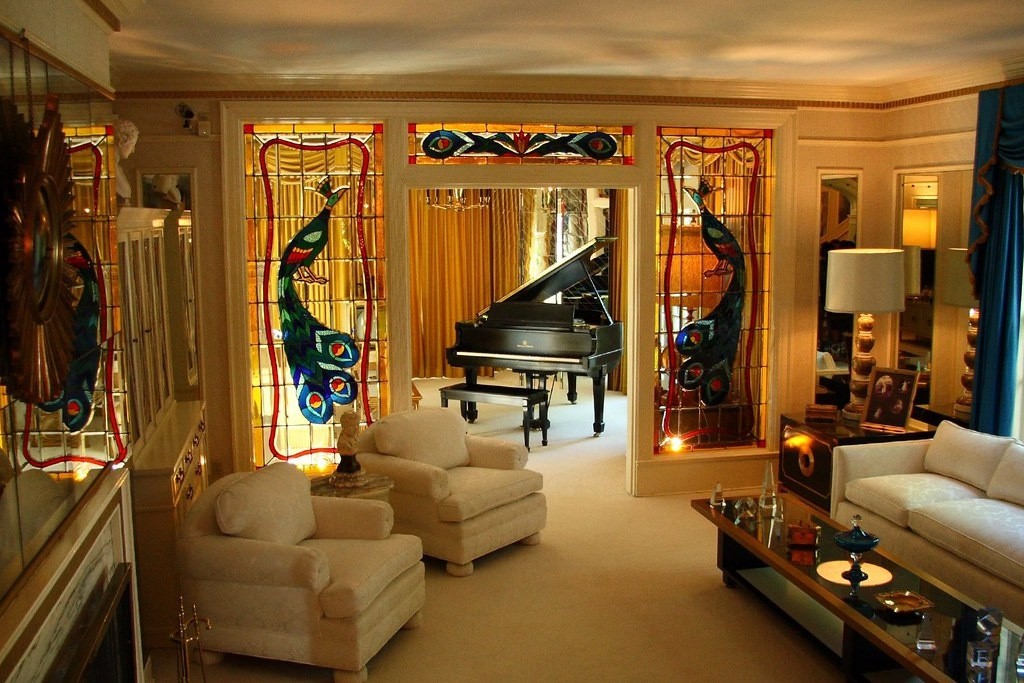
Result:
[804,404,838,420]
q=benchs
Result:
[439,383,550,452]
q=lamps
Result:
[657,209,725,407]
[423,188,492,213]
[823,247,906,422]
[940,247,982,412]
[174,103,195,129]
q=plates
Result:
[873,589,936,613]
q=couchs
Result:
[173,461,426,683]
[829,419,1024,629]
[357,405,547,577]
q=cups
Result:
[759,460,776,509]
[771,496,785,523]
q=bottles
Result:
[757,504,776,548]
[710,480,723,506]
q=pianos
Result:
[445,236,623,437]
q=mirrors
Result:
[133,166,205,403]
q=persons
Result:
[337,411,361,455]
[819,240,856,343]
[113,118,140,199]
[151,173,183,205]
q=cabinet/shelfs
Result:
[115,207,207,651]
[899,299,933,344]
[164,210,200,400]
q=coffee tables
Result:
[690,493,1024,683]
[312,473,394,503]
[777,411,926,518]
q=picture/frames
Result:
[859,365,920,434]
